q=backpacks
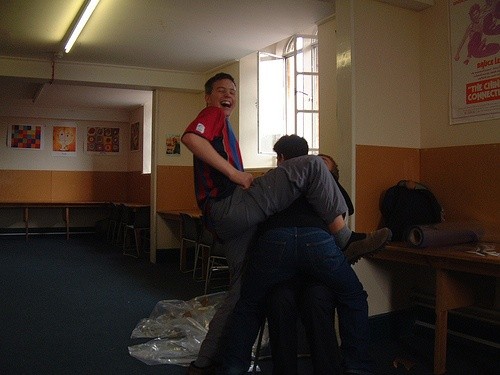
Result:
[377,180,441,242]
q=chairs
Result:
[178,212,230,295]
[106,202,150,258]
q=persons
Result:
[264,154,340,375]
[215,134,374,375]
[181,72,394,375]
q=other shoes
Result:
[185,361,216,375]
[343,228,392,264]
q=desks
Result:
[363,239,500,375]
[157,207,202,220]
[0,202,110,241]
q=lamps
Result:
[63,0,99,54]
